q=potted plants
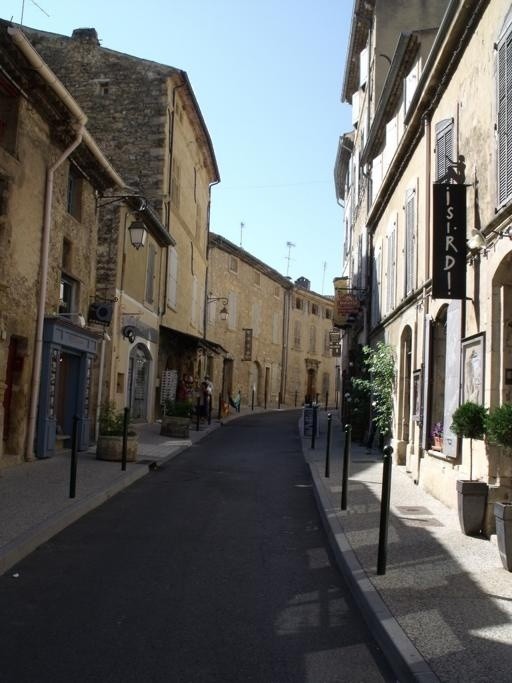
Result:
[158,396,194,439]
[448,401,512,573]
[91,394,141,463]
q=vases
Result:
[434,436,443,447]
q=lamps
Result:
[207,294,230,321]
[96,192,150,251]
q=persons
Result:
[200,374,213,390]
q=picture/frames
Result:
[411,369,422,421]
[460,331,486,410]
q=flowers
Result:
[430,421,444,438]
